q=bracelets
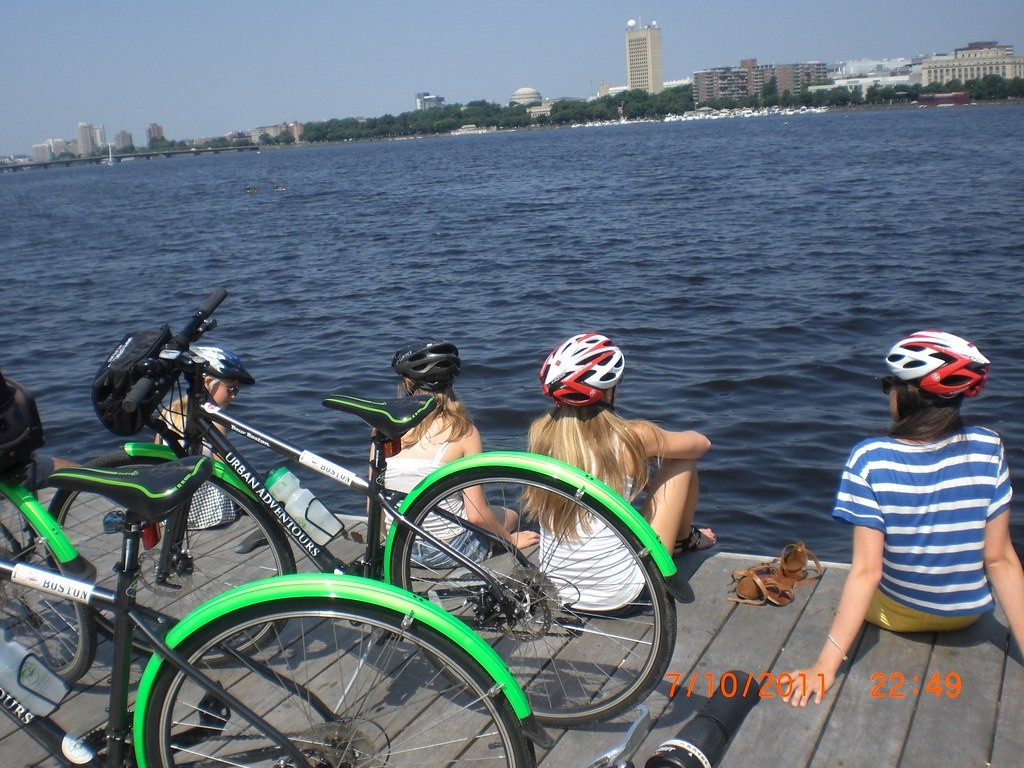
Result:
[827,634,849,662]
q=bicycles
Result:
[0,372,559,768]
[43,290,695,734]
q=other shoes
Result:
[103,510,127,533]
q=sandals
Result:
[727,541,820,606]
[673,524,717,557]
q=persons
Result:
[774,330,1024,707]
[366,335,541,569]
[519,333,716,616]
[30,454,82,488]
[154,347,257,530]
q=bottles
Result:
[0,628,69,718]
[263,466,344,547]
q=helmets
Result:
[391,335,461,389]
[539,332,626,408]
[884,329,991,398]
[184,341,255,384]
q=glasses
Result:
[340,529,365,544]
[220,380,239,396]
[881,376,907,395]
